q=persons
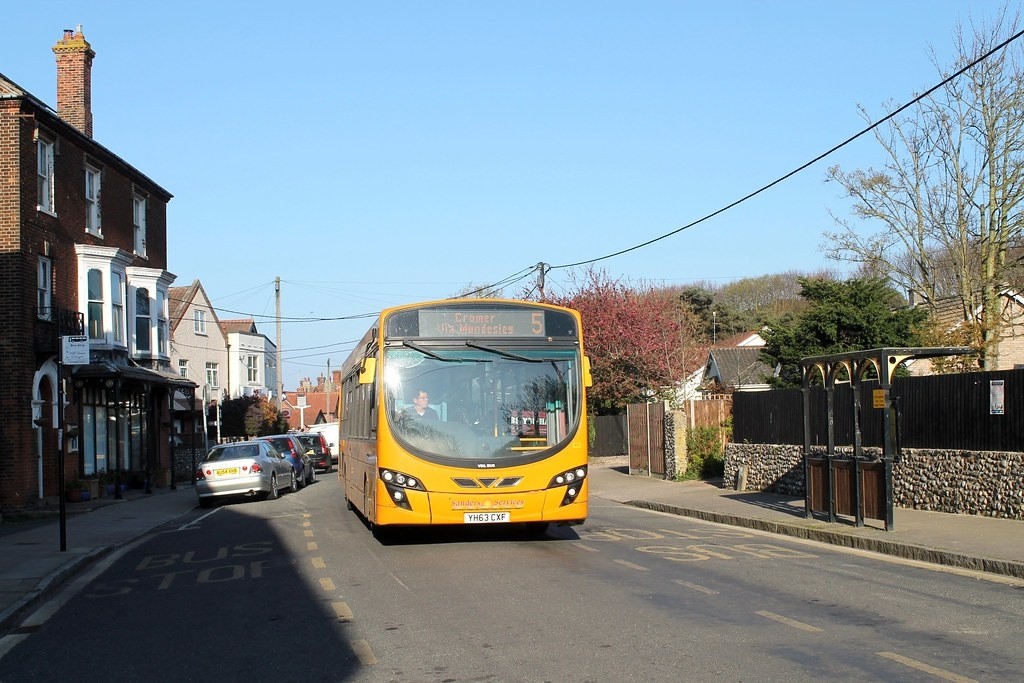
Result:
[399,389,438,419]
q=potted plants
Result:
[99,483,126,497]
[81,486,91,501]
[81,478,100,499]
[69,487,84,502]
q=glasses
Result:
[419,396,429,400]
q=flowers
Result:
[65,479,82,490]
[85,467,126,485]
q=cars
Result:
[256,433,316,487]
[194,440,299,503]
[289,432,334,474]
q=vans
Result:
[307,422,340,462]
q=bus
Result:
[332,297,595,532]
